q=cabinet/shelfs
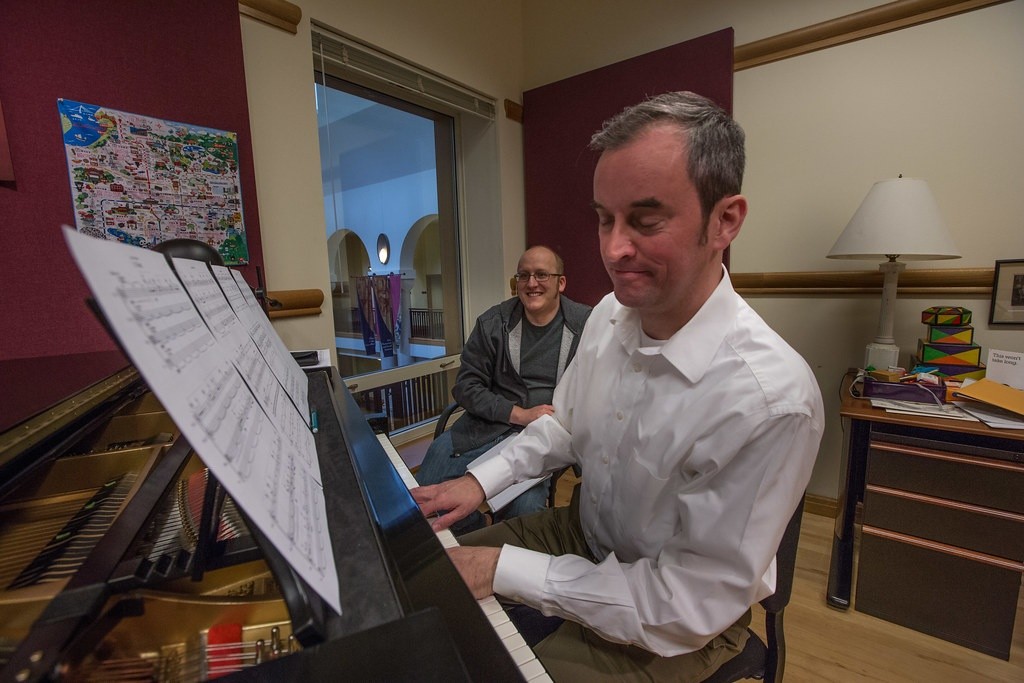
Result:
[825,374,1024,662]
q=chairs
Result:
[699,489,806,683]
[433,403,571,509]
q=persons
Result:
[414,246,593,537]
[409,91,825,683]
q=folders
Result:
[951,378,1024,415]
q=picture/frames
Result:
[988,259,1024,325]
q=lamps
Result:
[826,174,962,370]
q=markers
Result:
[311,405,319,433]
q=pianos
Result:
[0,343,557,683]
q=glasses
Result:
[514,269,560,282]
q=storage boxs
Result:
[863,372,947,404]
[945,386,970,402]
[911,354,987,383]
[916,338,982,368]
[927,325,974,345]
[870,369,900,382]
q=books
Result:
[951,378,1023,416]
